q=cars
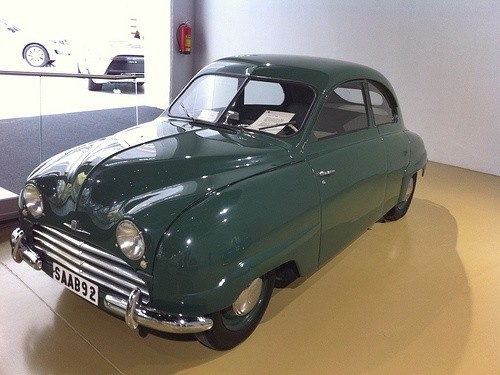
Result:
[13,29,144,91]
[9,54,428,352]
